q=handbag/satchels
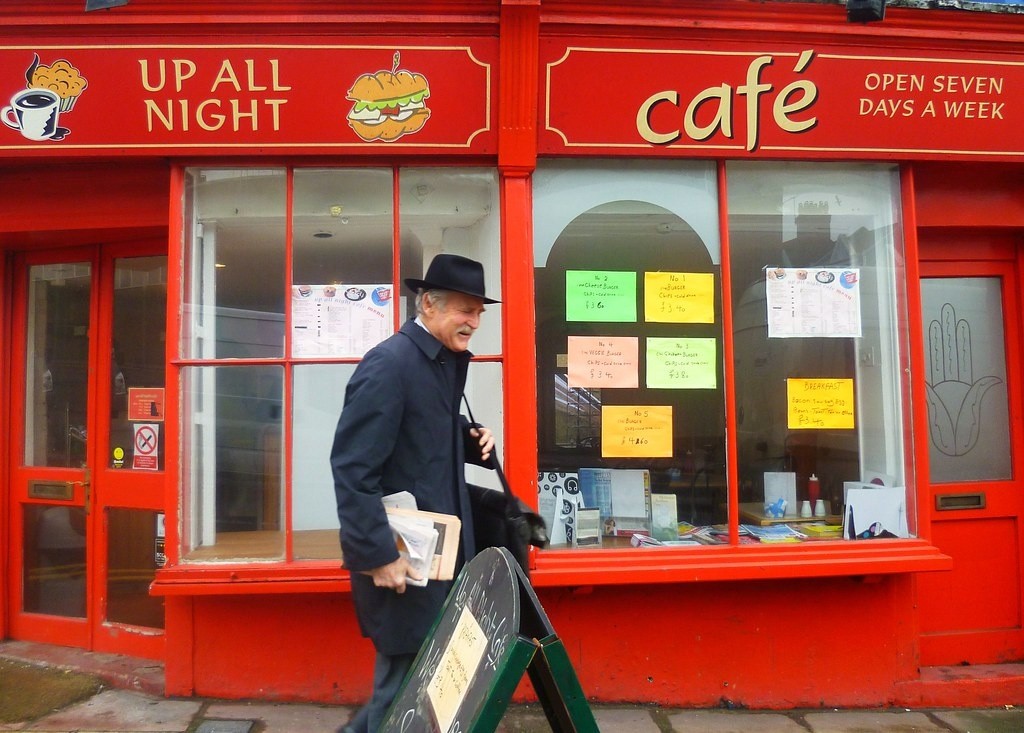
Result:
[467,483,549,583]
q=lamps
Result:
[845,0,885,24]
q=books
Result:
[359,490,462,589]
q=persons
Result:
[330,253,496,733]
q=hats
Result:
[405,254,504,304]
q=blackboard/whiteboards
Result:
[375,544,601,733]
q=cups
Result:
[802,501,812,519]
[815,500,825,517]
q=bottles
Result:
[686,449,694,475]
[831,496,841,516]
[809,473,821,513]
